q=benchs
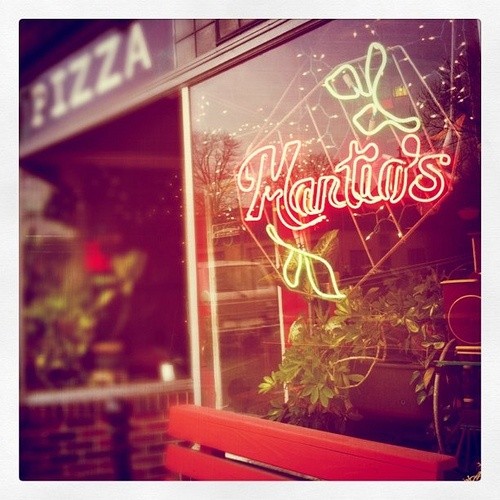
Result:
[162,404,456,481]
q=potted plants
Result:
[255,267,463,433]
